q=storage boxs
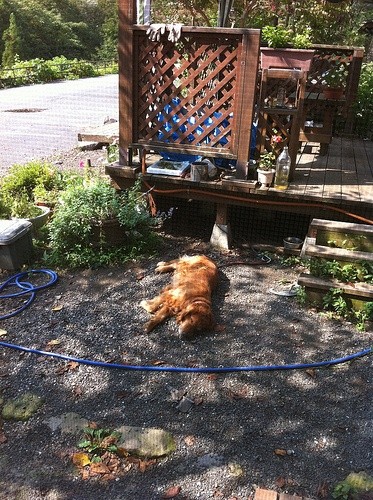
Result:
[0,219,35,272]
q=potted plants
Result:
[34,184,57,218]
[10,187,50,240]
[32,167,164,269]
[321,69,344,99]
[258,25,313,72]
[257,150,275,186]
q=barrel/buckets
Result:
[191,162,209,182]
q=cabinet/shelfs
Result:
[254,68,306,181]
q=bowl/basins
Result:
[282,236,303,249]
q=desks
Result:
[288,88,345,155]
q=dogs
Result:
[138,252,227,343]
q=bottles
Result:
[274,144,291,189]
